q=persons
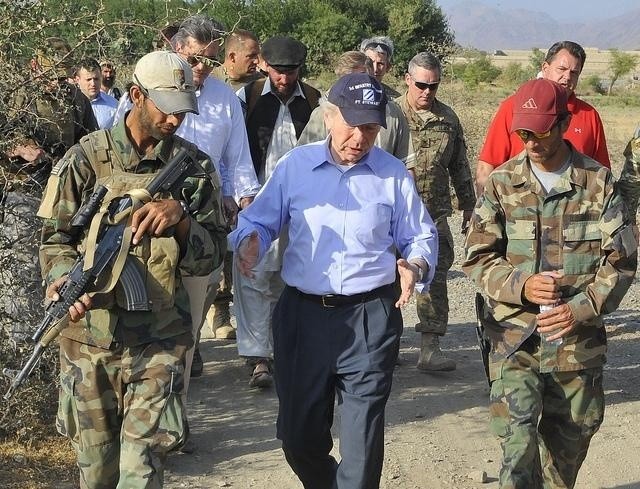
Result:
[461,81,637,489]
[619,120,640,230]
[300,50,418,184]
[395,52,478,372]
[230,74,439,489]
[359,35,402,97]
[2,14,319,489]
[475,40,611,195]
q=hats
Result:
[36,45,74,79]
[328,73,388,129]
[130,51,199,116]
[510,77,568,134]
[261,37,307,72]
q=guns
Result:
[2,144,212,403]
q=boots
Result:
[417,332,456,372]
[201,299,236,339]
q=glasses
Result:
[187,54,218,68]
[415,81,439,90]
[365,43,388,51]
[519,128,551,140]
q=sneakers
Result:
[191,347,204,376]
[248,358,273,388]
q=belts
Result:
[300,293,358,309]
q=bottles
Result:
[537,302,563,347]
